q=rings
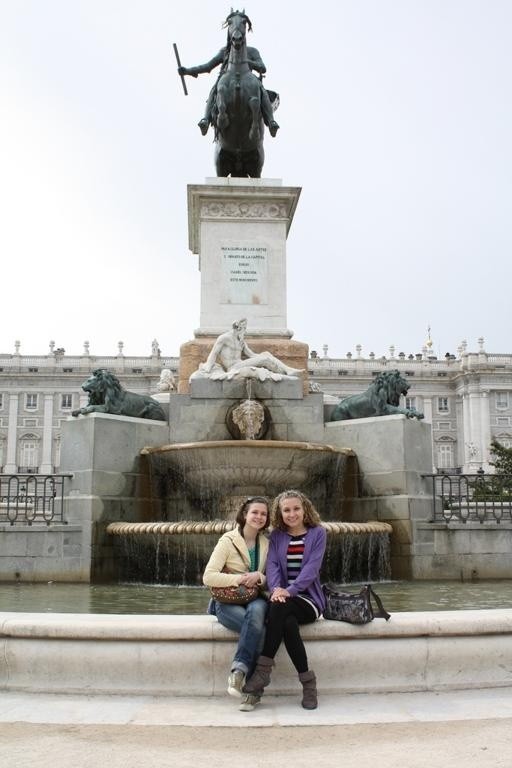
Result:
[246,581,249,584]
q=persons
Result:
[238,490,327,710]
[202,496,270,711]
[199,317,308,377]
[178,44,280,137]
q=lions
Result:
[330,369,424,421]
[71,368,167,421]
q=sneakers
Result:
[227,668,245,699]
[239,694,261,712]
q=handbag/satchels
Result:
[322,584,390,624]
[210,536,258,604]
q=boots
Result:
[298,669,317,710]
[242,655,275,693]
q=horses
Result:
[210,6,265,178]
